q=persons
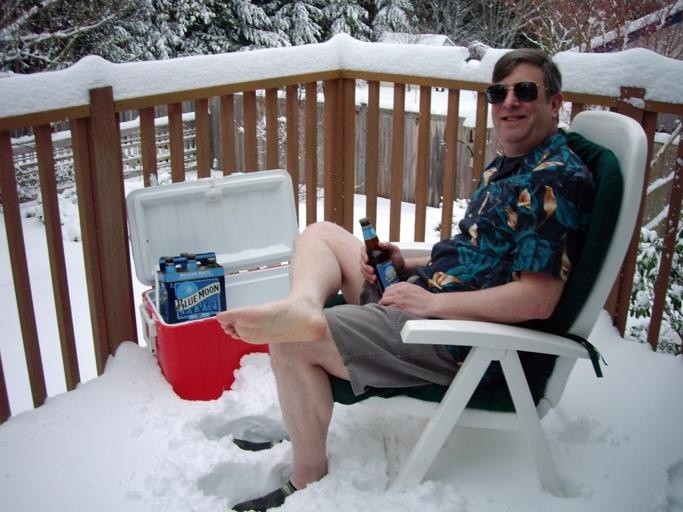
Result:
[216,48,597,512]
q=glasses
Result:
[486,81,547,104]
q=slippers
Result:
[232,480,298,512]
[234,435,290,451]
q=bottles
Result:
[360,218,400,295]
[160,251,226,272]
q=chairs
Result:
[359,112,650,496]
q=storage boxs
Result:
[124,168,298,401]
